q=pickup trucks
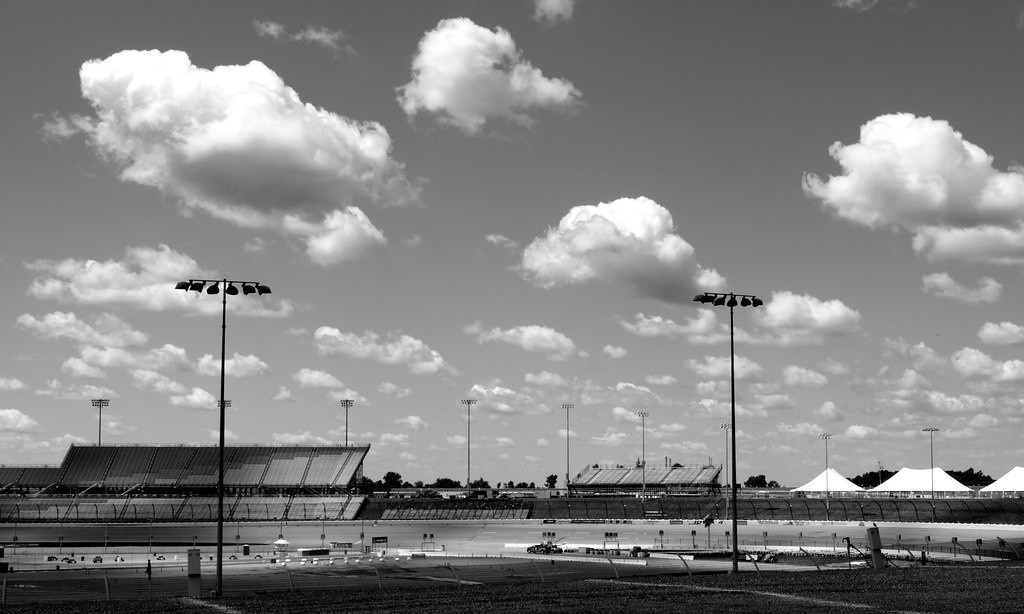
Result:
[526,544,551,555]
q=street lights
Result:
[561,403,575,498]
[922,426,939,499]
[462,399,478,495]
[819,433,831,510]
[720,422,732,520]
[177,277,274,599]
[340,399,355,447]
[637,411,650,501]
[691,291,764,579]
[92,398,111,447]
